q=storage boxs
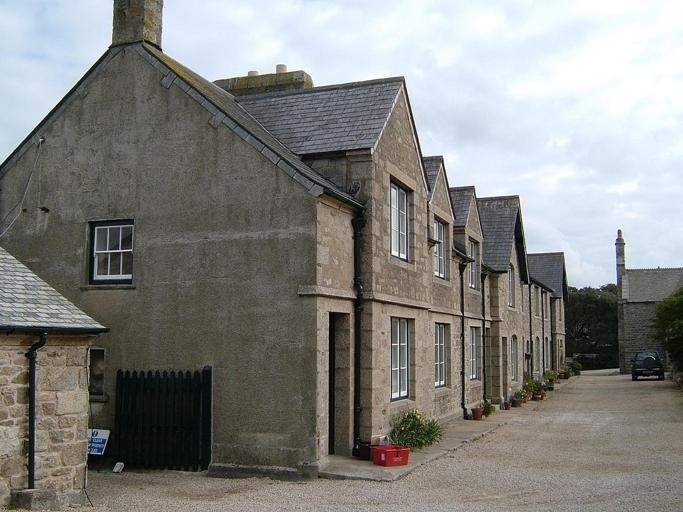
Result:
[370,445,410,466]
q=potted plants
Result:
[470,369,566,420]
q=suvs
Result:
[630,351,665,380]
